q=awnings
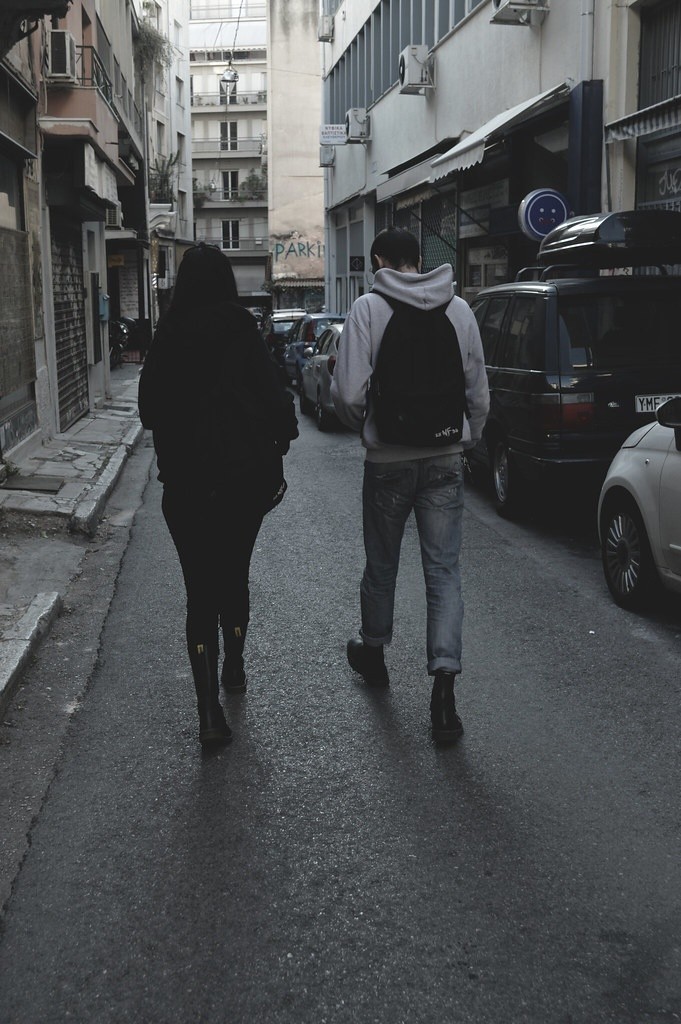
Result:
[534,209,681,262]
[427,81,569,187]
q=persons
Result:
[329,226,493,740]
[134,241,300,745]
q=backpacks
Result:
[370,286,472,447]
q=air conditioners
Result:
[48,30,76,84]
[344,107,366,143]
[320,146,334,165]
[317,14,334,41]
[106,201,123,229]
[398,44,429,93]
[491,0,538,22]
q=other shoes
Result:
[199,709,233,744]
[221,662,247,693]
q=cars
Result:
[300,323,347,434]
[247,306,350,388]
[597,395,681,612]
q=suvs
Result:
[467,207,680,522]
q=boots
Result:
[347,640,390,686]
[430,672,463,740]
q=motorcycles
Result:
[109,316,137,370]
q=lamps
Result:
[209,181,215,191]
